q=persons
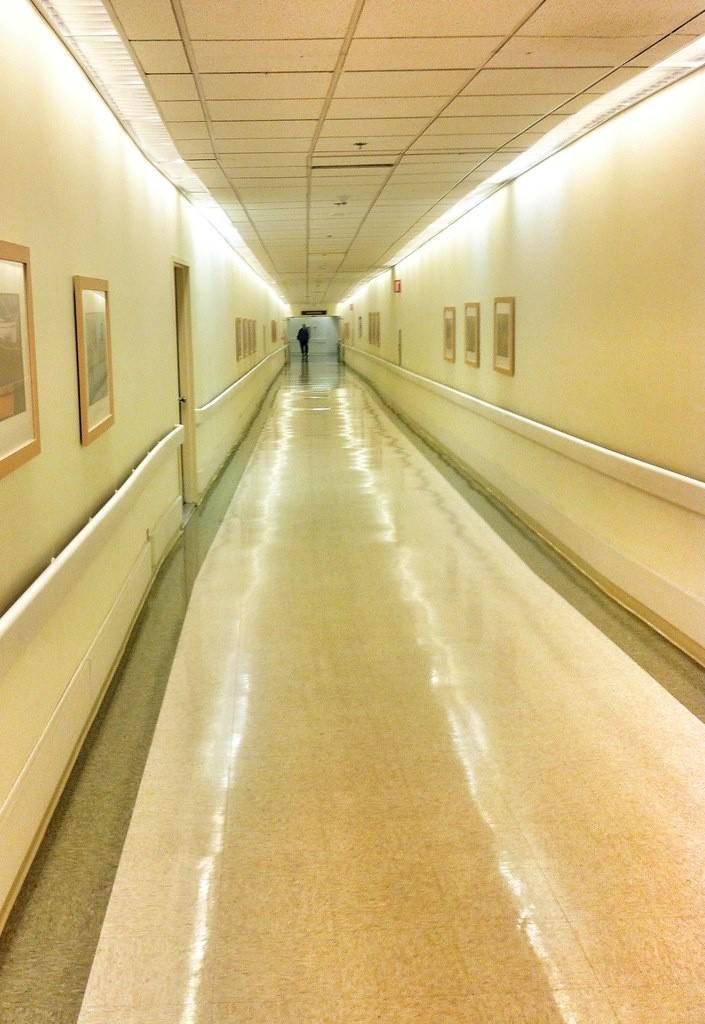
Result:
[297,323,309,356]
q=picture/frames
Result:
[72,274,116,448]
[492,296,516,377]
[464,302,479,369]
[443,306,457,364]
[0,239,41,480]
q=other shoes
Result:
[302,354,304,357]
[305,353,308,356]
